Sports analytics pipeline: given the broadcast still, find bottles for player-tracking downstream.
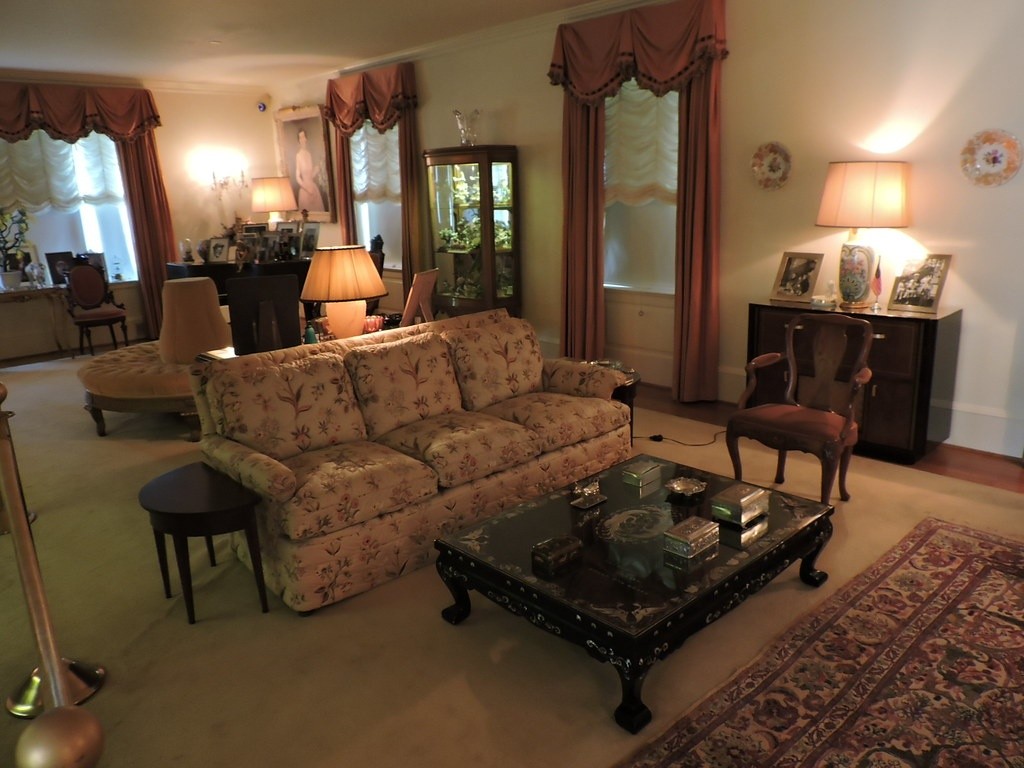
[305,325,317,344]
[197,240,211,262]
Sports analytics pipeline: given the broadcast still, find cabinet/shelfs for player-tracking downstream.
[747,301,962,465]
[420,144,522,319]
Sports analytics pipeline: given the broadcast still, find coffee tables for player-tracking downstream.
[612,519,1024,768]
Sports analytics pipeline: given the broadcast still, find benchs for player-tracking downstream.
[76,339,201,442]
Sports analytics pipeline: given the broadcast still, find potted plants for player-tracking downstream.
[0,207,28,290]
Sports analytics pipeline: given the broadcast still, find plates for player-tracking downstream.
[665,477,707,496]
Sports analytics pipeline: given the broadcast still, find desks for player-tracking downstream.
[431,450,835,734]
[167,261,314,357]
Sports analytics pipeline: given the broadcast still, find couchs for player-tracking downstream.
[190,309,633,616]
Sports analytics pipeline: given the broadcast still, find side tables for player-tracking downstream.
[594,358,641,447]
[138,459,268,624]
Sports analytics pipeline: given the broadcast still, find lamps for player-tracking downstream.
[815,162,909,306]
[251,177,299,223]
[300,245,388,340]
[156,277,227,369]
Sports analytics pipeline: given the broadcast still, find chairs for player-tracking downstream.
[725,312,873,506]
[61,254,129,355]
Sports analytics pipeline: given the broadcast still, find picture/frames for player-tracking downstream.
[272,104,336,223]
[399,268,438,327]
[227,274,301,356]
[768,251,824,303]
[238,221,321,261]
[208,237,230,262]
[887,254,952,314]
